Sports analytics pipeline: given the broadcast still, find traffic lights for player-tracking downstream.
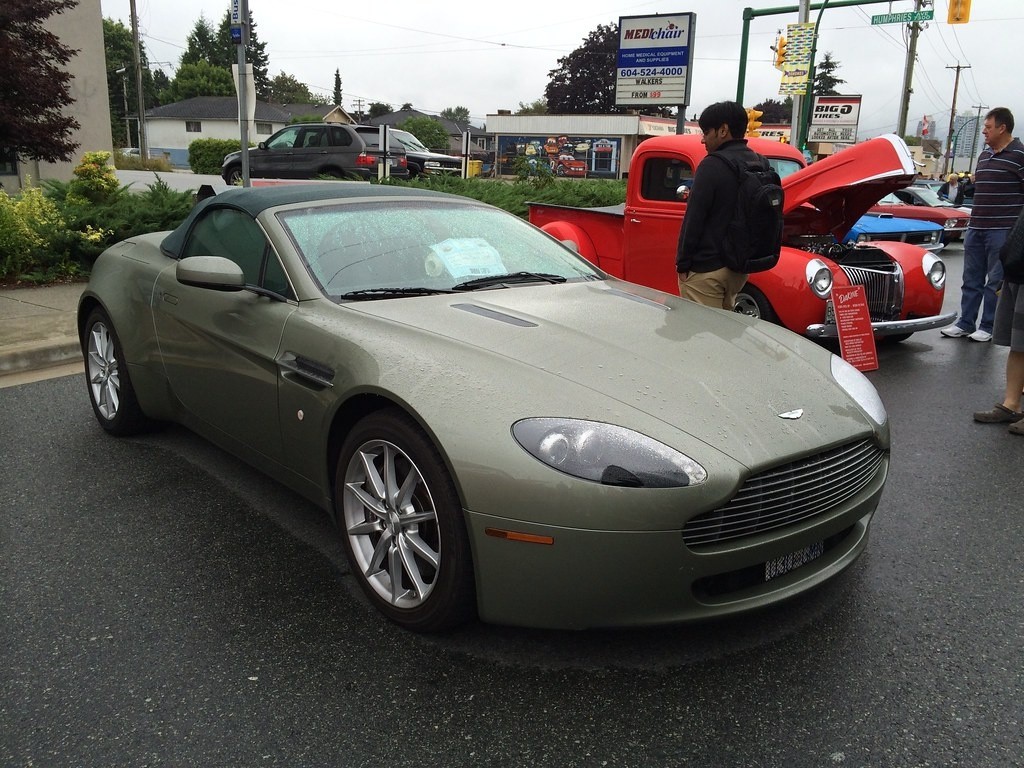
[747,110,763,138]
[775,35,788,70]
[780,137,786,142]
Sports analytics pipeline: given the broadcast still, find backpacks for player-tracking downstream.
[709,150,785,274]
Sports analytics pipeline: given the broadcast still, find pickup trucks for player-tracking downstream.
[522,132,959,340]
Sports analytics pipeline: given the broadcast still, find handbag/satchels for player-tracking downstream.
[996,204,1024,286]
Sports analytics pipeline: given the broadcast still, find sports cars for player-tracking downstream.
[76,181,893,635]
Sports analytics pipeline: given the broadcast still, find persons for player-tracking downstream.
[910,171,975,197]
[941,107,1024,344]
[937,174,964,205]
[974,209,1024,435]
[676,101,784,311]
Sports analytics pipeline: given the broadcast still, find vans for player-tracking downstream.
[947,173,972,182]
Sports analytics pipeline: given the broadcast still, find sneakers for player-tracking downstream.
[941,325,975,338]
[966,330,992,341]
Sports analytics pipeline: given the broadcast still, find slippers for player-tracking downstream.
[1008,417,1024,436]
[973,401,1023,421]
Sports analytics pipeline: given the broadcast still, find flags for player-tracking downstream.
[923,115,929,135]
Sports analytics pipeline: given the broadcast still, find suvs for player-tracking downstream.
[221,121,410,186]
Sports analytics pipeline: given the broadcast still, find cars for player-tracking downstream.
[912,179,973,204]
[119,148,151,159]
[834,216,944,256]
[867,193,970,248]
[894,187,972,226]
[307,126,462,178]
